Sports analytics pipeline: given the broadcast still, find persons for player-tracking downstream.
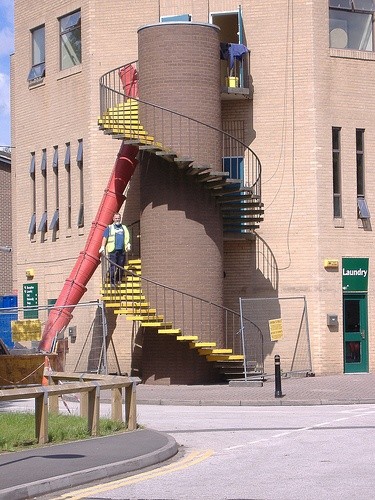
[98,213,131,287]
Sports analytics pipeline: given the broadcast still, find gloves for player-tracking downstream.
[126,243,131,251]
[99,245,105,253]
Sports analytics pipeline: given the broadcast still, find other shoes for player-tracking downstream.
[117,280,122,284]
[112,284,115,287]
